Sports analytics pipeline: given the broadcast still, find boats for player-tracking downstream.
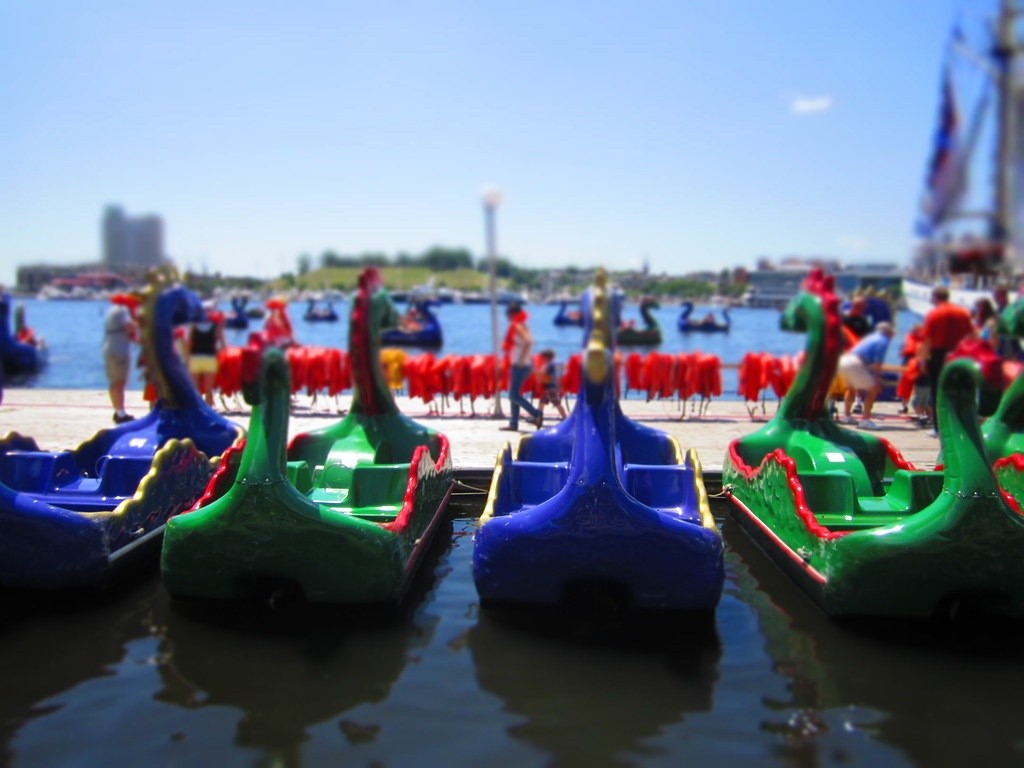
[1,266,902,388]
[722,263,1023,625]
[160,267,452,629]
[0,267,247,614]
[472,265,727,629]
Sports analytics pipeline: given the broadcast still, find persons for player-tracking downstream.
[499,303,544,431]
[839,322,897,429]
[100,286,145,424]
[704,312,714,322]
[525,348,569,426]
[897,284,1024,437]
[184,310,228,406]
[623,318,637,333]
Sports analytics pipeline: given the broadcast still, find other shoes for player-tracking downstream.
[113,413,133,423]
[535,416,542,430]
[841,416,858,424]
[858,420,881,429]
[526,417,536,423]
[500,426,517,431]
